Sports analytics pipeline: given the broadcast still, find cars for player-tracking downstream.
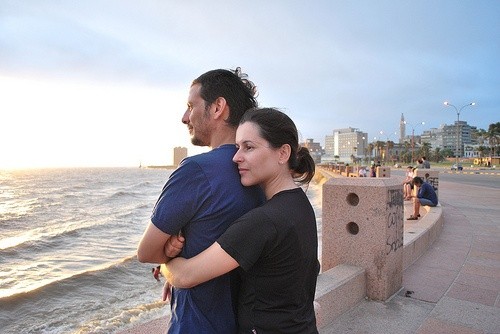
[451,165,462,171]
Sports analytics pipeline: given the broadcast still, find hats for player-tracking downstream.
[408,166,413,168]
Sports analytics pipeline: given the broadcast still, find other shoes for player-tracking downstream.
[407,217,418,220]
[411,214,420,217]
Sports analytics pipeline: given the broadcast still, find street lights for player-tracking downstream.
[403,122,425,166]
[444,102,476,165]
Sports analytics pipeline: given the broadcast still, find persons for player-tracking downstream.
[407,176,438,220]
[359,156,430,201]
[154,265,163,281]
[159,106,321,334]
[137,66,267,334]
